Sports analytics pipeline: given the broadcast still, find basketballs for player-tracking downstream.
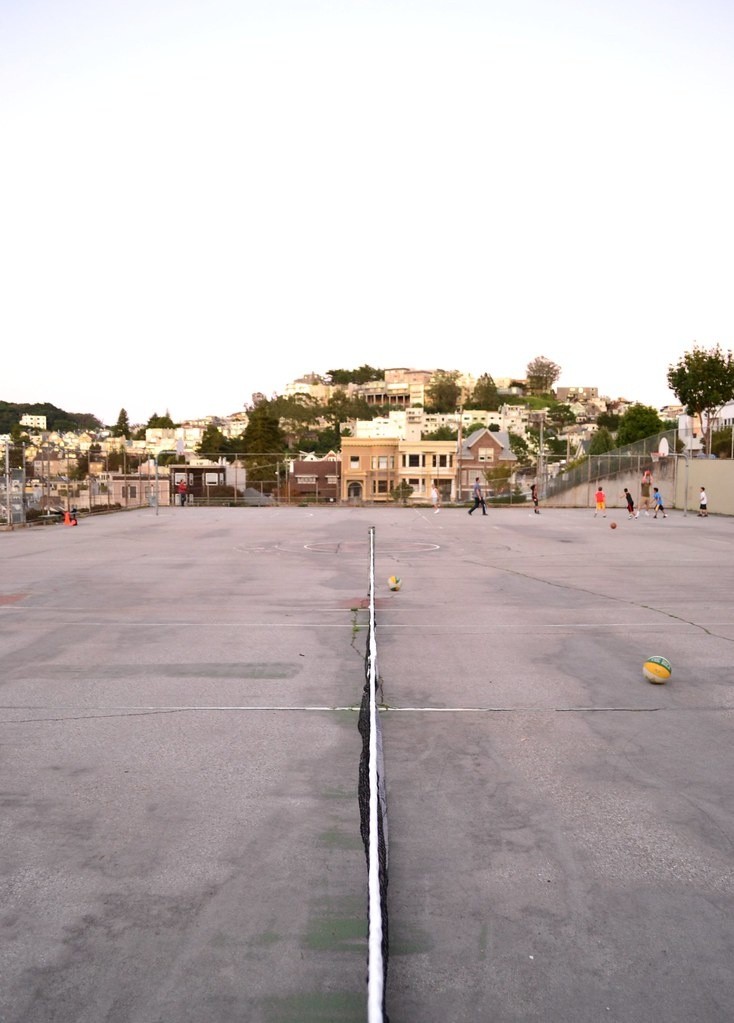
[611,522,616,529]
[643,656,672,685]
[645,471,651,476]
[387,576,402,591]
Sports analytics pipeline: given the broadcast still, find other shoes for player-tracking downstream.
[482,513,488,515]
[636,511,640,517]
[468,511,473,516]
[703,514,708,517]
[663,515,667,518]
[602,514,606,517]
[435,508,440,513]
[697,514,702,517]
[634,516,638,520]
[644,510,649,516]
[628,516,631,520]
[652,515,657,518]
[534,510,541,514]
[593,513,597,517]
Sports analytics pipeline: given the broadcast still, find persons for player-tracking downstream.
[431,484,440,514]
[621,488,636,519]
[530,485,539,514]
[636,471,653,516]
[697,487,708,518]
[651,488,667,518]
[179,479,188,506]
[595,487,606,518]
[468,477,488,516]
